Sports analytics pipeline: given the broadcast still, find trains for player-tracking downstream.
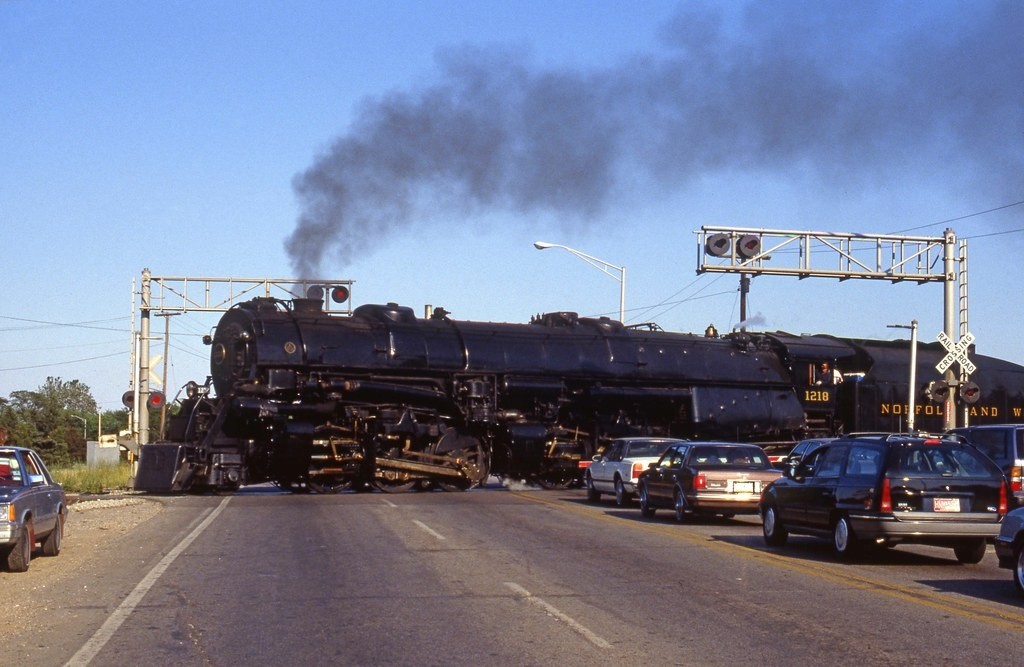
[134,294,1022,493]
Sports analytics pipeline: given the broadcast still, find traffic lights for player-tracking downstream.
[307,285,349,303]
[122,388,166,409]
[707,232,761,258]
[927,379,981,404]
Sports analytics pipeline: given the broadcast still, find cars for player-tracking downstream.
[586,436,688,505]
[0,446,67,573]
[636,441,783,523]
[781,438,840,474]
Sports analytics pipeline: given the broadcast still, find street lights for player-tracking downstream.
[71,414,87,440]
[534,241,626,325]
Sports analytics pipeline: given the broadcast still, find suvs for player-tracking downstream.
[759,431,1009,565]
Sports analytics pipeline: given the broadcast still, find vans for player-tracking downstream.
[937,424,1023,511]
